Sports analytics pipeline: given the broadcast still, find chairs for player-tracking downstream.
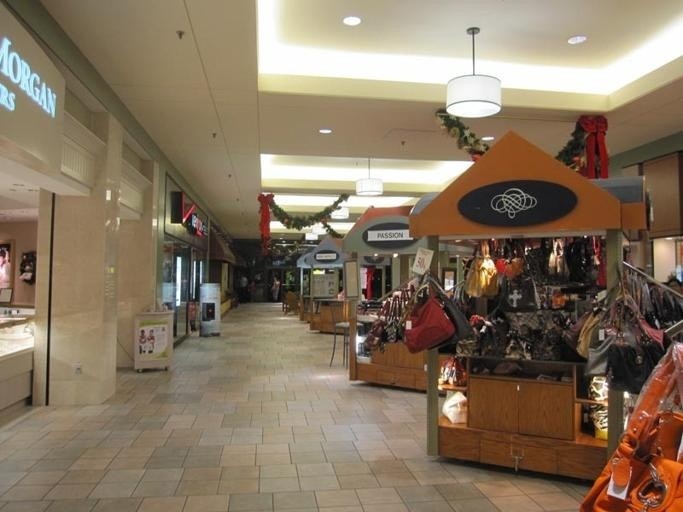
[281,290,301,314]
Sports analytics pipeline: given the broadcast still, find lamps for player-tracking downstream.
[445,27,502,118]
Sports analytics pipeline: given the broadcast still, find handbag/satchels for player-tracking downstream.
[403,273,474,355]
[456,236,683,392]
[579,343,683,511]
[365,279,416,350]
[440,390,468,425]
[438,357,466,387]
